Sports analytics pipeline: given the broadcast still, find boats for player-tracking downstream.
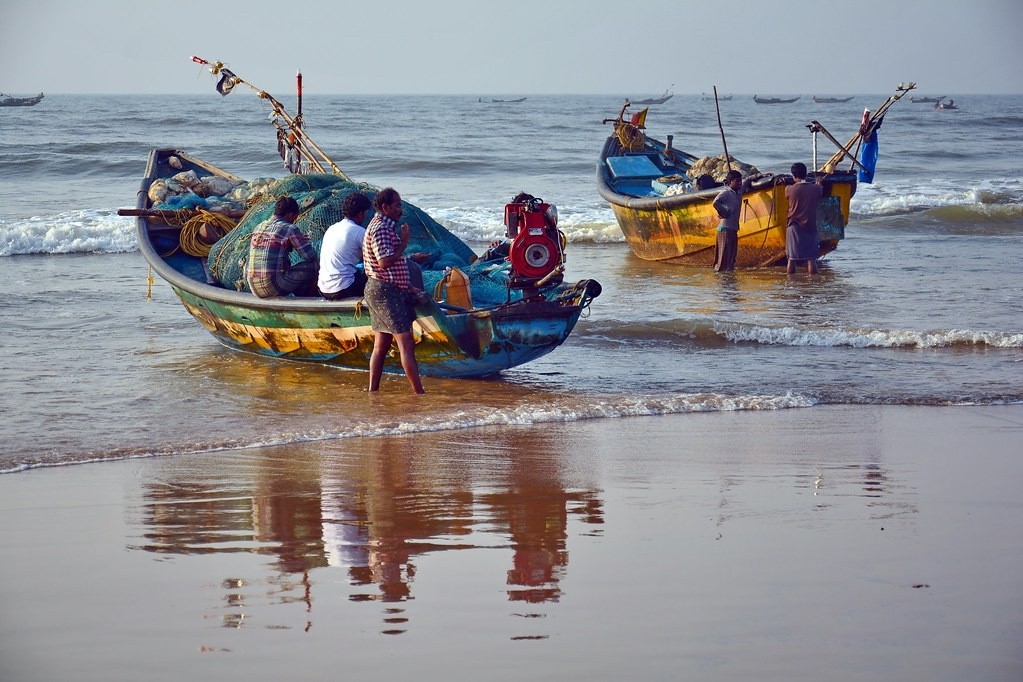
[594,78,922,268]
[811,96,856,103]
[492,97,528,103]
[935,99,959,110]
[0,92,47,108]
[703,94,734,101]
[115,53,605,380]
[752,94,802,104]
[909,96,949,104]
[625,90,676,105]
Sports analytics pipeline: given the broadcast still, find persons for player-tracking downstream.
[315,195,433,302]
[784,162,825,275]
[249,197,320,299]
[712,170,774,273]
[363,188,427,395]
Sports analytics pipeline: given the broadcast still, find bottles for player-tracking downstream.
[437,266,473,314]
